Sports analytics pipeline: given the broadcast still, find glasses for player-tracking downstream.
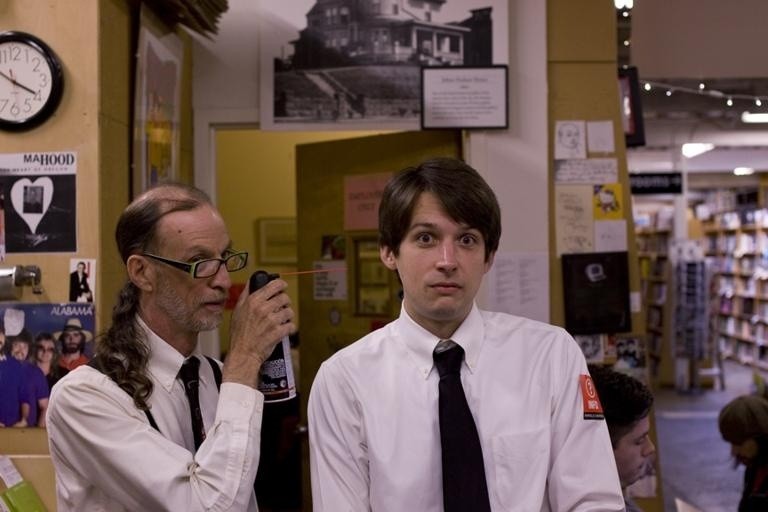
[140,250,249,279]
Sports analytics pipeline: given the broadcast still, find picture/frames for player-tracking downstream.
[420,65,508,130]
[257,216,298,265]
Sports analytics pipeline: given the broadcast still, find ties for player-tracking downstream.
[176,356,207,452]
[432,346,490,512]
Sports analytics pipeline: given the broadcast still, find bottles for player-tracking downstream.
[247,270,297,403]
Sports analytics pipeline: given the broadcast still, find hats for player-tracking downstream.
[719,396,768,440]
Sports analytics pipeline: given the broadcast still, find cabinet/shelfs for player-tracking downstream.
[637,225,768,393]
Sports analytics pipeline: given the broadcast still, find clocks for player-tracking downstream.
[0,30,64,133]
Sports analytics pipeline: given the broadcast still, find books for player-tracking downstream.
[635,184,768,391]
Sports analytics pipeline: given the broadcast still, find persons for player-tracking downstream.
[717,394,768,512]
[306,157,625,512]
[0,319,90,430]
[589,360,657,512]
[70,262,94,302]
[45,181,296,511]
